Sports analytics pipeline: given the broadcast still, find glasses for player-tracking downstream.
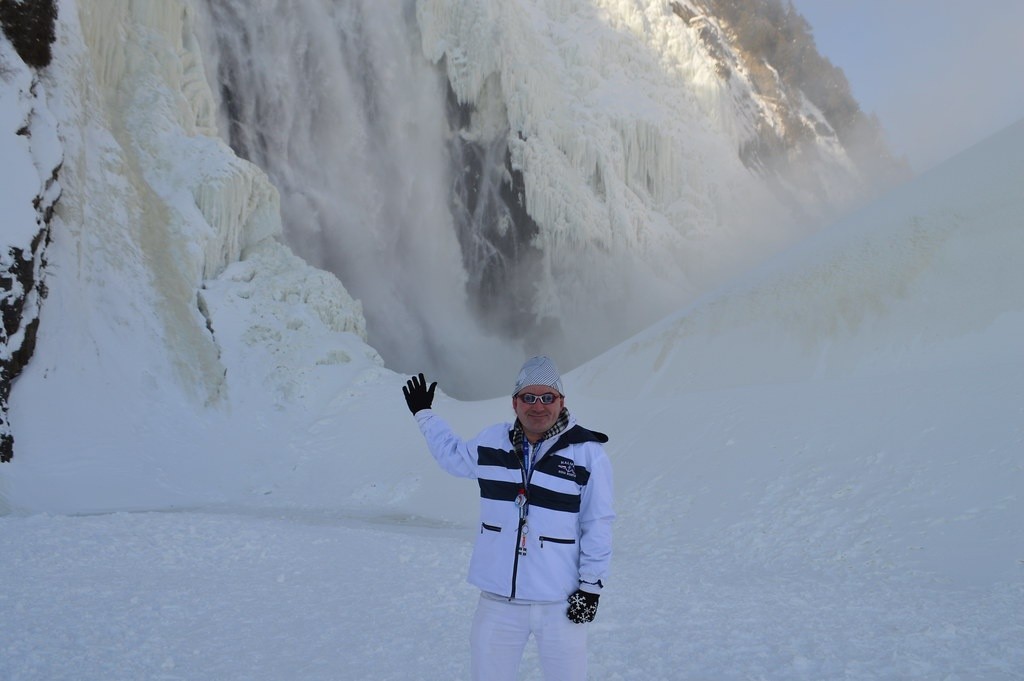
[517,393,560,404]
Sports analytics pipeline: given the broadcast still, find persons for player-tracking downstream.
[402,354,616,681]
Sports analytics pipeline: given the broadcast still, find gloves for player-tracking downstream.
[403,372,437,416]
[567,590,600,623]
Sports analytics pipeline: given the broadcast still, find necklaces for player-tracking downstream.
[514,435,542,556]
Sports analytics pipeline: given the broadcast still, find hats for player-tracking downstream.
[512,355,565,397]
